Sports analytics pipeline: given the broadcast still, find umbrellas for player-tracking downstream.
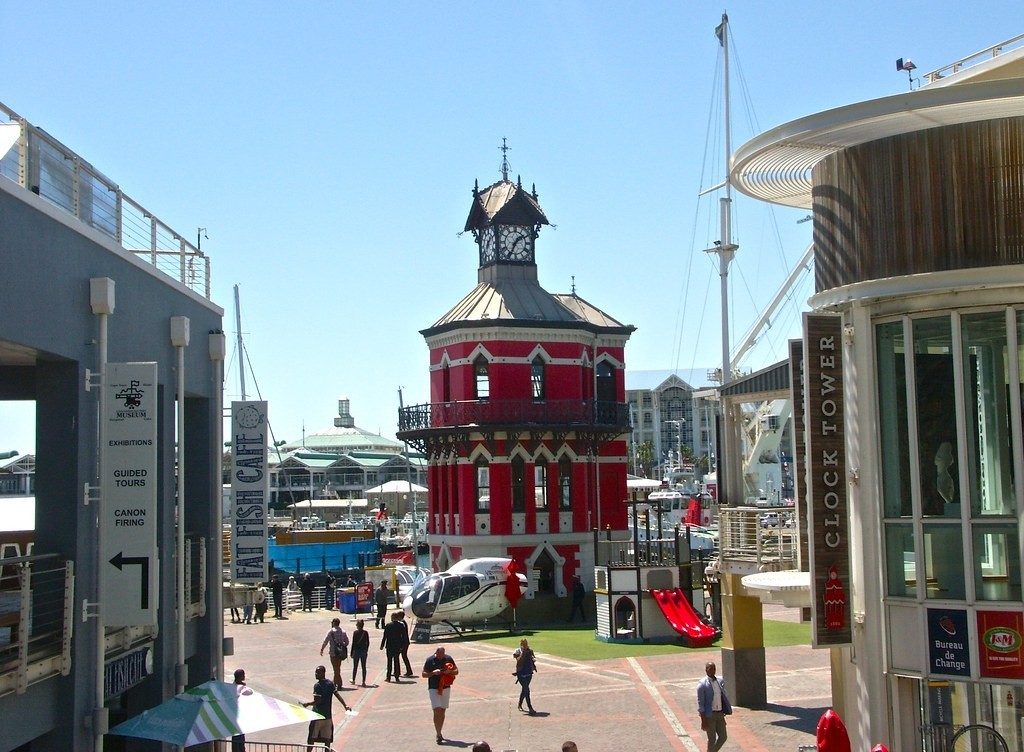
[104,678,325,752]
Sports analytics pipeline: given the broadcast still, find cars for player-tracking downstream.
[334,518,413,530]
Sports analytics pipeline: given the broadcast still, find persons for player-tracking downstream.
[232,570,355,624]
[514,638,537,714]
[935,442,954,504]
[375,580,389,629]
[351,620,369,686]
[422,646,458,745]
[380,612,405,682]
[473,740,492,752]
[392,612,413,676]
[566,575,586,622]
[320,618,349,689]
[563,741,578,752]
[303,666,351,752]
[697,662,727,752]
[231,669,245,752]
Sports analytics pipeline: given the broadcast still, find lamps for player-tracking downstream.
[896,57,920,91]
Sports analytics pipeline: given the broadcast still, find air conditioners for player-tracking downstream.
[763,414,777,433]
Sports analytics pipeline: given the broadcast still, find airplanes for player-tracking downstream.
[410,557,540,643]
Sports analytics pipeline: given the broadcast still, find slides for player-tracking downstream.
[649,587,716,639]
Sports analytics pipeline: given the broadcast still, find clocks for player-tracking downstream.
[497,223,533,263]
[480,225,497,265]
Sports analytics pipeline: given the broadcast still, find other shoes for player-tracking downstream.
[337,686,342,690]
[404,672,412,676]
[529,710,536,714]
[350,679,354,684]
[383,626,386,629]
[396,678,399,682]
[384,678,389,682]
[436,734,442,743]
[518,705,523,711]
[375,623,379,629]
[362,683,365,687]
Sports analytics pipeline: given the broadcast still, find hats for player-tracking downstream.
[331,618,340,623]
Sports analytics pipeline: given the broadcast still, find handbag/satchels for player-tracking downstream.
[335,643,347,659]
